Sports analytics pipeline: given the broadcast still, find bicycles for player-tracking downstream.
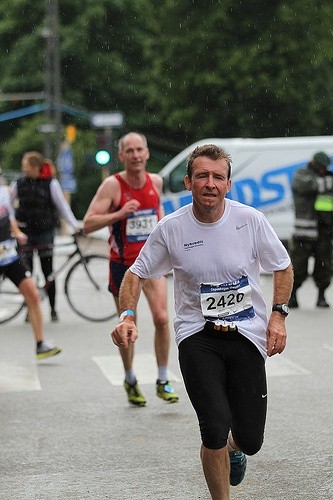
[0,229,118,324]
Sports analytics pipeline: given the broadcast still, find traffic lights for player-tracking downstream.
[96,149,111,165]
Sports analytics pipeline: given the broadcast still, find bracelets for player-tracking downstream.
[119,309,136,323]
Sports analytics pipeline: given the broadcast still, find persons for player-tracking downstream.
[289,152,333,309]
[82,132,180,406]
[6,151,84,322]
[109,144,293,500]
[0,164,60,360]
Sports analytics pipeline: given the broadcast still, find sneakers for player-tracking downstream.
[155,379,179,403]
[228,449,246,487]
[36,345,61,360]
[123,376,146,407]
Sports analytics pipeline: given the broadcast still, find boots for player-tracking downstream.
[317,289,330,306]
[288,287,298,307]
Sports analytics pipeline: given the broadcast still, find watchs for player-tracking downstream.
[272,303,290,317]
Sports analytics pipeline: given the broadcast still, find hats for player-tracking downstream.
[313,152,331,165]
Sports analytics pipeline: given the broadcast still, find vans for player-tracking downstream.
[156,135,332,245]
